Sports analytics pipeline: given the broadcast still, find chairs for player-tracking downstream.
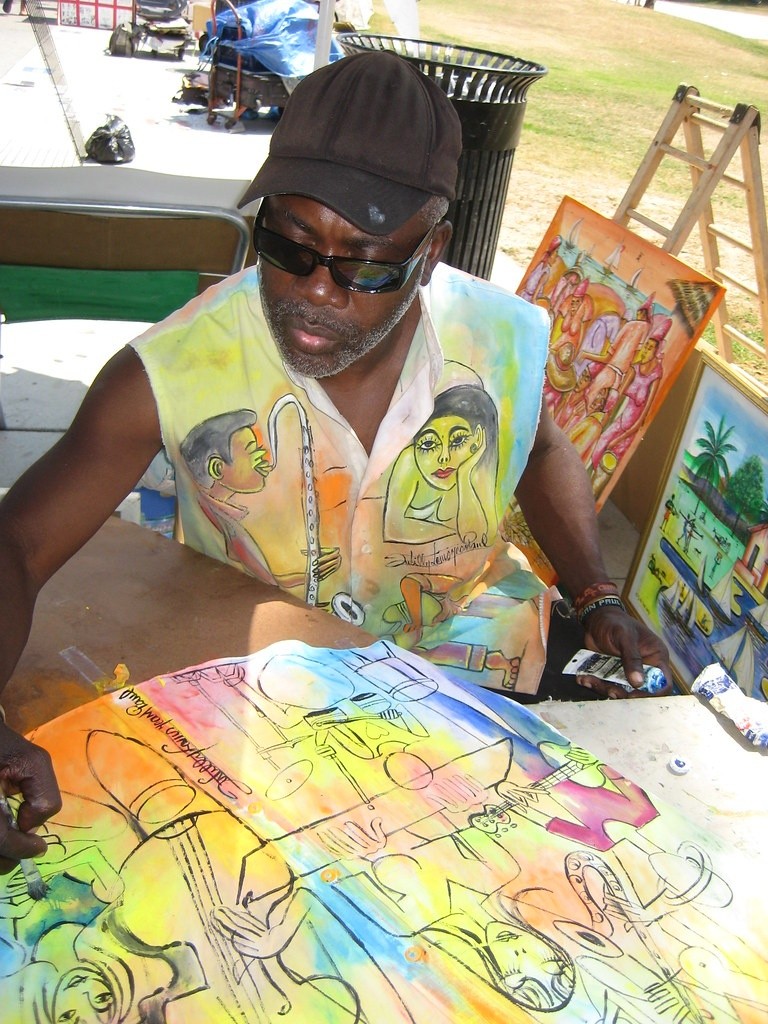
[0,198,253,344]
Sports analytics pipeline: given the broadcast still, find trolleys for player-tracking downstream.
[132,0,187,61]
[207,0,248,130]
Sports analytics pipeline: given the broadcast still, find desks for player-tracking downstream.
[1,491,683,1024]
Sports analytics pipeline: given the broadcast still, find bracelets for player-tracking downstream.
[571,582,622,624]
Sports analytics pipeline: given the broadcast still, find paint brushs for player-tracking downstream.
[0,784,52,902]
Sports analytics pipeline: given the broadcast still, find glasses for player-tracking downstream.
[253,196,436,294]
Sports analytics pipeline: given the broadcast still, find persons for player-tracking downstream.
[0,49,675,878]
[2,0,27,16]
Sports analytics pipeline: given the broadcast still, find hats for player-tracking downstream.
[238,50,463,236]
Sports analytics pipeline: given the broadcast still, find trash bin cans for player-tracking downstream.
[338,31,550,281]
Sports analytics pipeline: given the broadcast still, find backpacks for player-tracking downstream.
[109,23,135,57]
